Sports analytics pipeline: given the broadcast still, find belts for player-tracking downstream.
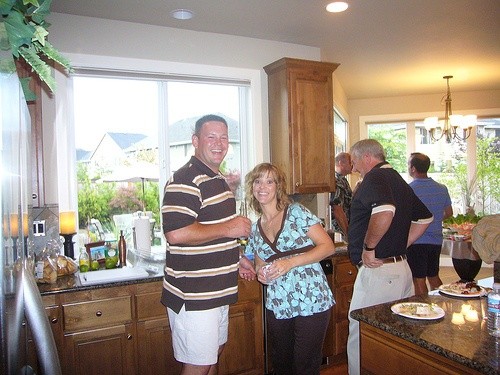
[357,254,406,269]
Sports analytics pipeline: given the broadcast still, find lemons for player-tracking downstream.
[79,258,116,272]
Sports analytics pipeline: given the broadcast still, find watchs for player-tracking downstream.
[362,242,375,251]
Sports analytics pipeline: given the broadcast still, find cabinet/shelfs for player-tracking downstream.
[263,57,341,193]
[6,253,359,375]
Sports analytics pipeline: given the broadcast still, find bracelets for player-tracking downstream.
[239,254,245,260]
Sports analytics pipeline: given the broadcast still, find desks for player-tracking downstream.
[350,276,500,375]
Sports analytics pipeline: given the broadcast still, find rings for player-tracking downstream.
[277,270,280,274]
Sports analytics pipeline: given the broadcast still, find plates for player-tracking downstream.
[439,283,486,298]
[391,303,445,320]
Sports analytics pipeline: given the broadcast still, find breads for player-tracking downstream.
[33,255,78,283]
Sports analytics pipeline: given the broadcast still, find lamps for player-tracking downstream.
[423,76,476,142]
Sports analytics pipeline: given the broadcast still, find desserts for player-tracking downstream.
[456,279,480,293]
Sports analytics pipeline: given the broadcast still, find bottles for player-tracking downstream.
[488,283,500,338]
[77,230,128,272]
[238,201,249,245]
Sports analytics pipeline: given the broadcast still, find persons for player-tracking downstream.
[160,115,256,375]
[241,163,336,375]
[346,138,434,375]
[329,151,354,244]
[407,153,453,296]
